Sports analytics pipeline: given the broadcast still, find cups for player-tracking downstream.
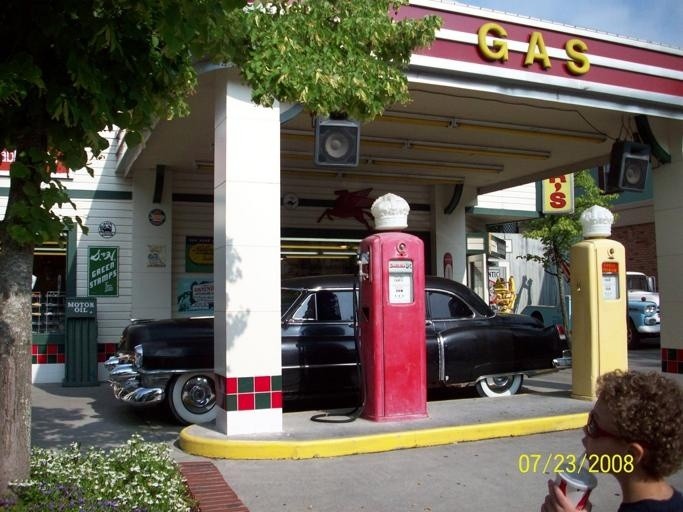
[555,463,598,512]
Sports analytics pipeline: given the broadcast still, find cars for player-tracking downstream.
[105,274,573,425]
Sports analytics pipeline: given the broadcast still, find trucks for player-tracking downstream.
[520,271,661,350]
[179,282,214,311]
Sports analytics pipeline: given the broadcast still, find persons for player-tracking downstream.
[541,368,683,512]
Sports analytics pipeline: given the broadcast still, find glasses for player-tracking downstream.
[583,415,619,442]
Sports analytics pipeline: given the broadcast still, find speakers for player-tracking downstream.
[314,111,361,167]
[607,137,651,193]
